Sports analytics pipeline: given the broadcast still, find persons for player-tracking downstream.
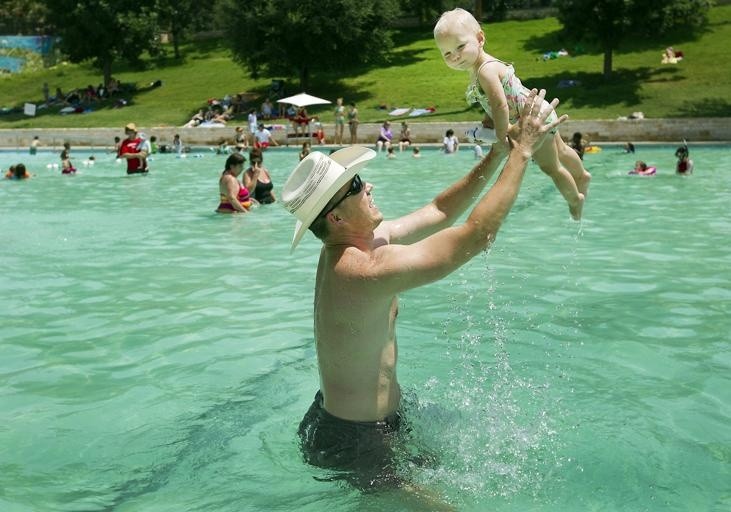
[661,47,684,63]
[280,86,571,512]
[627,158,654,177]
[215,151,254,217]
[536,46,571,60]
[673,148,692,175]
[241,147,278,206]
[433,8,592,219]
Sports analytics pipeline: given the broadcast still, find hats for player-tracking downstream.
[124,123,138,134]
[276,144,381,257]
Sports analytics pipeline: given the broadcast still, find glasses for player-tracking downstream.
[322,172,367,219]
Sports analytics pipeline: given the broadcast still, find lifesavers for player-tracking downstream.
[584,146,601,154]
[628,166,656,176]
[318,129,325,142]
